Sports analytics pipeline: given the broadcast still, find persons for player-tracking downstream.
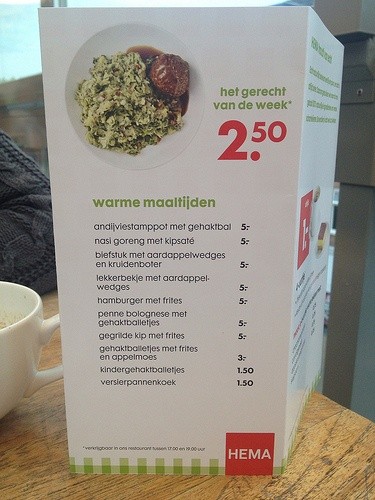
[0,127,56,298]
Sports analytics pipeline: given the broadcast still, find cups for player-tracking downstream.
[1,281,67,421]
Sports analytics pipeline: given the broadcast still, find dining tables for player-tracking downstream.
[0,280,375,500]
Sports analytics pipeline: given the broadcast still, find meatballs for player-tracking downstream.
[150,54,190,98]
